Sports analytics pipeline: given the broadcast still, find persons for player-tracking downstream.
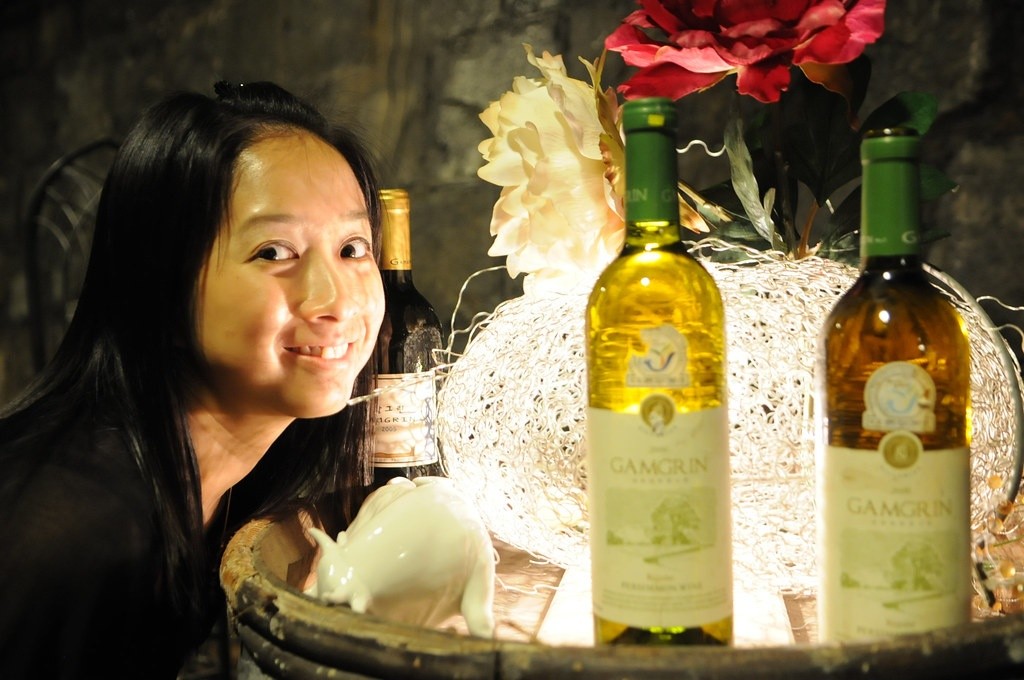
[0,82,388,680]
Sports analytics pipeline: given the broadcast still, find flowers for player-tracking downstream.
[476,0,937,295]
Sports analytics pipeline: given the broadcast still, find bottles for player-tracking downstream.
[816,128,973,642]
[340,189,445,486]
[584,98,734,644]
